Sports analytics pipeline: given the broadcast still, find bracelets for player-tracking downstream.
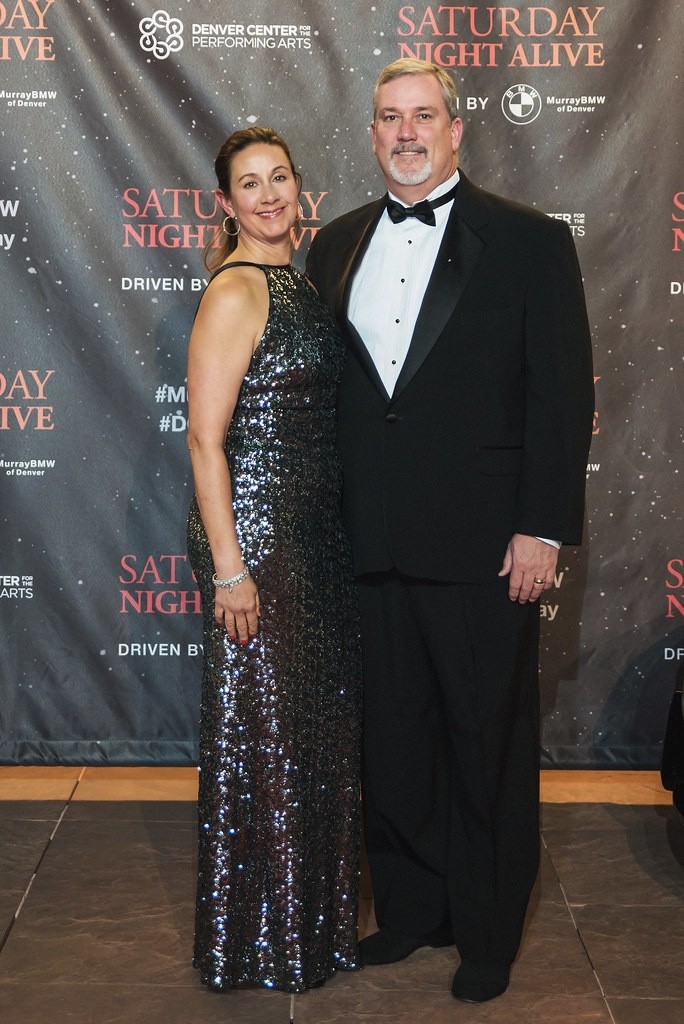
[213,565,250,593]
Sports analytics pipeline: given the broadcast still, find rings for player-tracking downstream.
[534,578,546,584]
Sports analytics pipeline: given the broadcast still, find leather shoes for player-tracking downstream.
[451,960,511,1004]
[355,925,424,966]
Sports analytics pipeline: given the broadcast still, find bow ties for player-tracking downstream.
[384,180,460,226]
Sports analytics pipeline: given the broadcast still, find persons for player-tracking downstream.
[308,59,594,1001]
[185,128,361,991]
